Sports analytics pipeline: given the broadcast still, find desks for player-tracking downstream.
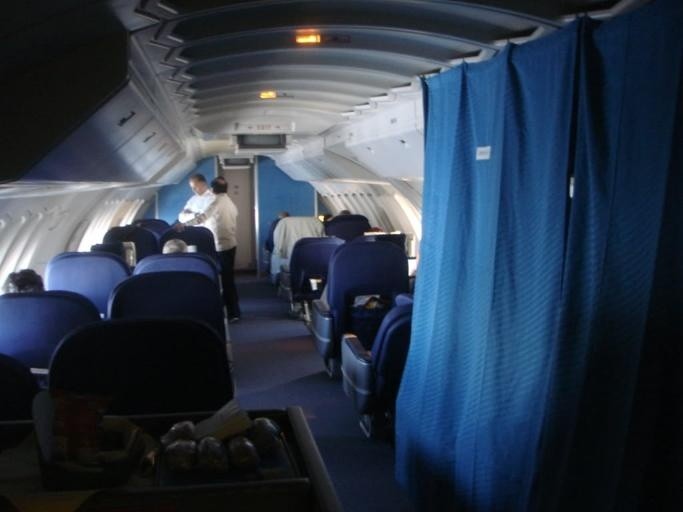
[0,407,344,512]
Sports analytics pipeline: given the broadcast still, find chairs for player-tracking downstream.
[308,236,408,378]
[339,302,413,437]
[159,224,216,252]
[103,225,154,259]
[269,215,325,285]
[275,236,346,306]
[134,217,169,234]
[132,252,219,288]
[0,352,41,450]
[325,215,370,240]
[47,313,235,418]
[0,289,100,386]
[44,252,129,318]
[105,270,228,346]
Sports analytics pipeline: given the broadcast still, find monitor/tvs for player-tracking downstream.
[224,159,249,166]
[236,135,286,149]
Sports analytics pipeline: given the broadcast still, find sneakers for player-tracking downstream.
[228,317,241,326]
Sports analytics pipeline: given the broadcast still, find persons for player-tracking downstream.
[3,269,43,294]
[162,238,188,254]
[178,174,216,228]
[173,176,240,323]
[268,212,289,250]
